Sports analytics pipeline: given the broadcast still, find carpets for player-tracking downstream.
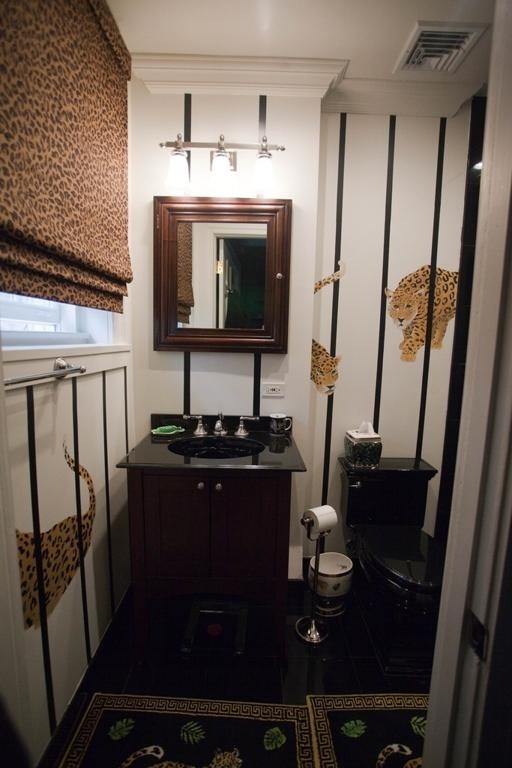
[40,691,316,767]
[305,692,431,767]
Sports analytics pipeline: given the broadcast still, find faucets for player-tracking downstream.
[212,410,229,437]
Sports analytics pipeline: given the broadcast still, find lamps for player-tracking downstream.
[158,131,287,193]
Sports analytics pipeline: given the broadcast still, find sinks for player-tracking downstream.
[167,436,267,460]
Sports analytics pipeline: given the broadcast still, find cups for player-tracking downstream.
[268,414,294,437]
[269,435,292,455]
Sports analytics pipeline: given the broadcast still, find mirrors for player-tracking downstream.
[150,192,293,355]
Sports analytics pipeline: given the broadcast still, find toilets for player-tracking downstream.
[336,454,439,665]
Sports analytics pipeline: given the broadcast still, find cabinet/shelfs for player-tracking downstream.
[115,424,308,646]
